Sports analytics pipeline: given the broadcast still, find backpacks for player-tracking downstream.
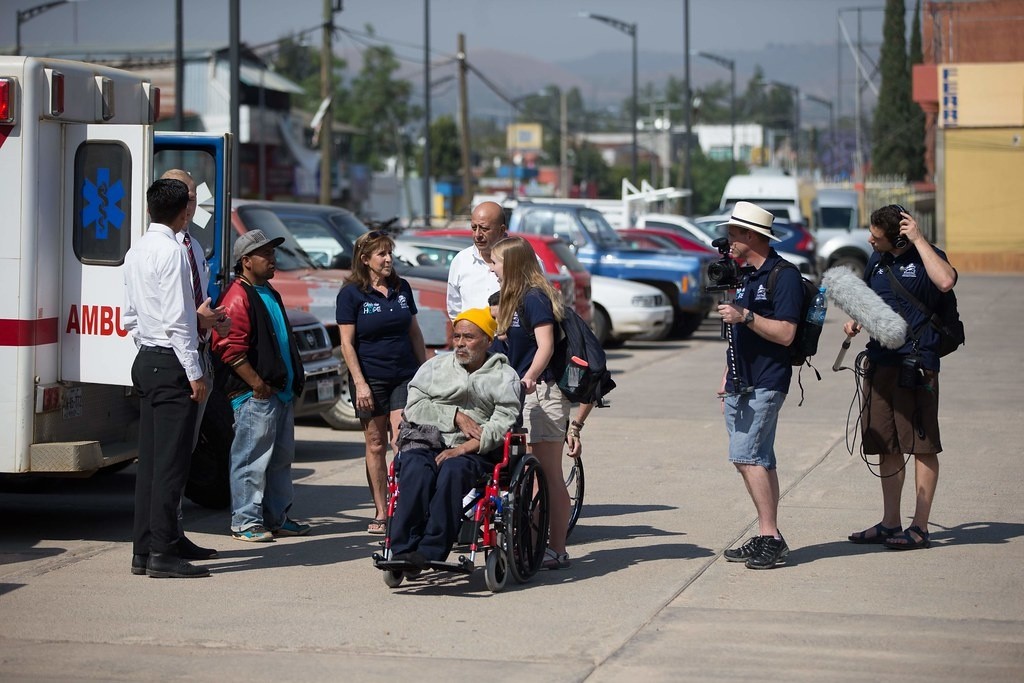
[560,306,617,403]
[765,258,828,368]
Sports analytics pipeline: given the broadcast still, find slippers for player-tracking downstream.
[367,517,388,534]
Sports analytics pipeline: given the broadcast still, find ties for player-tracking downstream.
[183,233,208,338]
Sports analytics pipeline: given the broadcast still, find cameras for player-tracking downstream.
[707,237,757,284]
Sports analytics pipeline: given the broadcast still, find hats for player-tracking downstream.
[452,306,498,341]
[715,201,782,243]
[232,229,286,261]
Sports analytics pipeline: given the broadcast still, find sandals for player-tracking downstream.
[884,525,930,550]
[848,521,903,544]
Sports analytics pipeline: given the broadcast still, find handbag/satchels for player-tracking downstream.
[883,250,965,359]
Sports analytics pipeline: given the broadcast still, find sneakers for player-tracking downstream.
[232,526,273,542]
[539,547,573,571]
[273,517,312,536]
[724,529,790,569]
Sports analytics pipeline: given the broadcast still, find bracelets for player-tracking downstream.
[567,421,584,436]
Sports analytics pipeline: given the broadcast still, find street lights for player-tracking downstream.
[801,93,835,182]
[689,49,737,175]
[759,79,800,168]
[422,0,457,226]
[578,12,639,195]
[11,0,70,56]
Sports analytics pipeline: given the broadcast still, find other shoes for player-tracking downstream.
[131,552,210,578]
[178,535,218,560]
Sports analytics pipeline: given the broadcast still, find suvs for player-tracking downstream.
[259,200,450,282]
[188,197,453,431]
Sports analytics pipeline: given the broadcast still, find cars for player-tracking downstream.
[294,172,878,350]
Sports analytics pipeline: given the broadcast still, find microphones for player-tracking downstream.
[822,264,909,350]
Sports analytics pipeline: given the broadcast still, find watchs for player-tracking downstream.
[745,310,754,325]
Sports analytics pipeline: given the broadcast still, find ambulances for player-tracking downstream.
[0,55,236,481]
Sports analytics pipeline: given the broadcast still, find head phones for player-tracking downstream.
[893,203,908,249]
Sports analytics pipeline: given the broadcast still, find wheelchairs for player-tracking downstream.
[371,381,586,593]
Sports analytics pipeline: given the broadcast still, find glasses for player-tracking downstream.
[361,230,389,255]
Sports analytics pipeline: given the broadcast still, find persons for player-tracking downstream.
[719,200,800,570]
[845,205,960,552]
[484,289,594,458]
[122,170,569,580]
